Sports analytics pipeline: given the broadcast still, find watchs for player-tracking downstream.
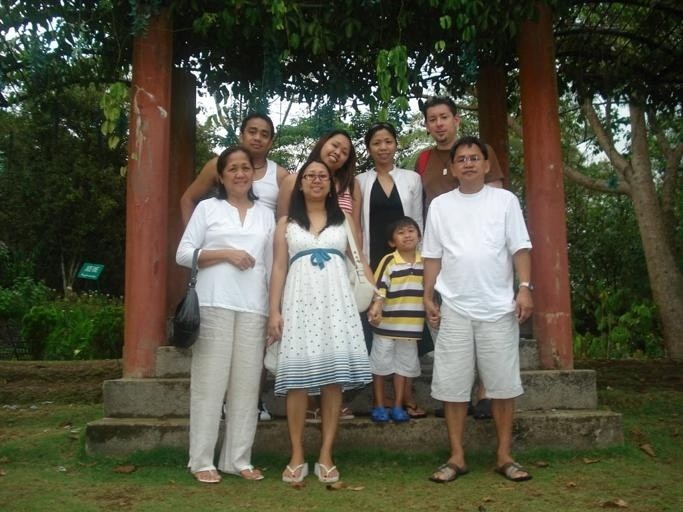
[517,280,536,293]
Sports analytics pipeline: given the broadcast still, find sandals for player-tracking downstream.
[194,469,221,483]
[240,465,264,480]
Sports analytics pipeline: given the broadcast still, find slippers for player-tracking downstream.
[372,405,426,421]
[304,407,322,424]
[430,461,468,483]
[496,460,532,481]
[340,407,354,421]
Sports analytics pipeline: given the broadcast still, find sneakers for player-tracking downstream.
[220,402,226,421]
[258,399,274,422]
[435,398,493,419]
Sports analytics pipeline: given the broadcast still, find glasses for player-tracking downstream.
[425,94,450,105]
[369,123,392,131]
[301,173,330,181]
[456,155,480,165]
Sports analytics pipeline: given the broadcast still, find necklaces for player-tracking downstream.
[247,157,267,175]
[434,147,456,185]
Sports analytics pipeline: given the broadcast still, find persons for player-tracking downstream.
[265,159,376,486]
[277,129,366,424]
[412,98,501,421]
[182,114,289,424]
[421,138,536,484]
[367,218,427,427]
[175,147,271,482]
[357,121,427,418]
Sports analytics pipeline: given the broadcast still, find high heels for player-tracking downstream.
[282,461,309,483]
[314,462,339,483]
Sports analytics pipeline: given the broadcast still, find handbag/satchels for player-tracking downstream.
[168,248,200,347]
[342,209,375,313]
